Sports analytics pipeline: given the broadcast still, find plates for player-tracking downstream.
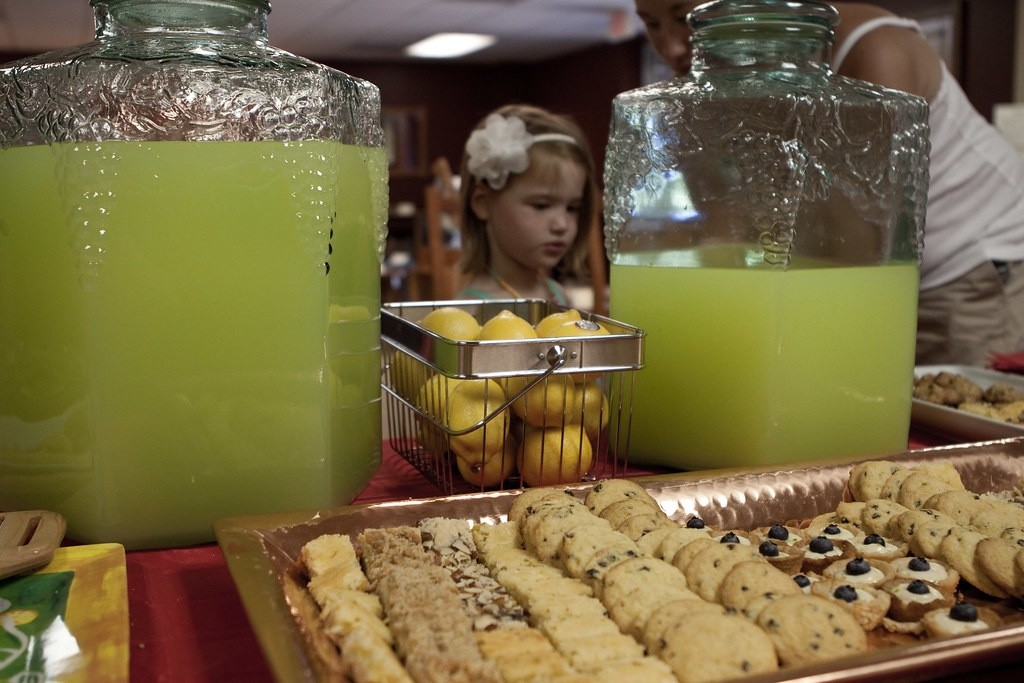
[215,436,1024,683]
[0,542,130,683]
[912,364,1024,442]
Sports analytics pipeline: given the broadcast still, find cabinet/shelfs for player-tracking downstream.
[408,182,605,313]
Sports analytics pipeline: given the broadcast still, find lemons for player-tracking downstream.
[391,307,614,490]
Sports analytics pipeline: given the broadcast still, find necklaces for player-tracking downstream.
[487,266,521,299]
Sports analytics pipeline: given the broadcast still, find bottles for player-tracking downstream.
[0,0,388,551]
[602,0,931,470]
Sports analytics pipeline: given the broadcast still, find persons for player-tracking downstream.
[635,0,1024,365]
[457,105,593,305]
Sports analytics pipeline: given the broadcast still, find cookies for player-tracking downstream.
[910,371,1024,427]
[295,457,1024,683]
